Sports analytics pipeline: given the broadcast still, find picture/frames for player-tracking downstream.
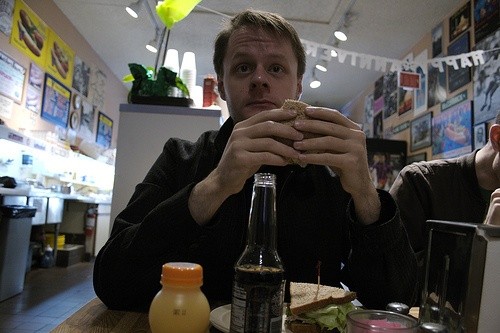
[373,0,500,177]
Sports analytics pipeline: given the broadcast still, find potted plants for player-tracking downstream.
[122,0,201,108]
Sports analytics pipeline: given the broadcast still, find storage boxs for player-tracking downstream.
[55,243,85,267]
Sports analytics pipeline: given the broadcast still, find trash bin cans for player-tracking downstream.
[0,205,37,302]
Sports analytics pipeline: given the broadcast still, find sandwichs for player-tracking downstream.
[285,282,364,333]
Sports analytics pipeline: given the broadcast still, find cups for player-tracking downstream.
[163,48,204,109]
[346,310,421,333]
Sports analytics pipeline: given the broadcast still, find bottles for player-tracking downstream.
[229,173,286,333]
[149,262,210,333]
[386,302,409,316]
[42,244,54,268]
[421,322,448,333]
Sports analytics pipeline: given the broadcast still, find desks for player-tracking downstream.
[0,186,112,261]
[46,296,228,333]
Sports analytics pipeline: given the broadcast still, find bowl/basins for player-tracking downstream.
[51,185,70,194]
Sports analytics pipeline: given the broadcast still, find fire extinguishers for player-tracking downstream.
[84,207,95,237]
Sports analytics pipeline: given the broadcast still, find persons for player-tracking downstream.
[91,11,424,322]
[471,42,500,112]
[384,112,500,308]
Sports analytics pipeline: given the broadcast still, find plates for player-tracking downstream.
[210,303,290,333]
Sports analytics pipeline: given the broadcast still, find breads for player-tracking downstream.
[272,100,315,167]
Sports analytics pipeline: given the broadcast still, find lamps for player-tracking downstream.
[307,39,339,89]
[126,0,146,20]
[145,28,161,53]
[333,12,353,41]
